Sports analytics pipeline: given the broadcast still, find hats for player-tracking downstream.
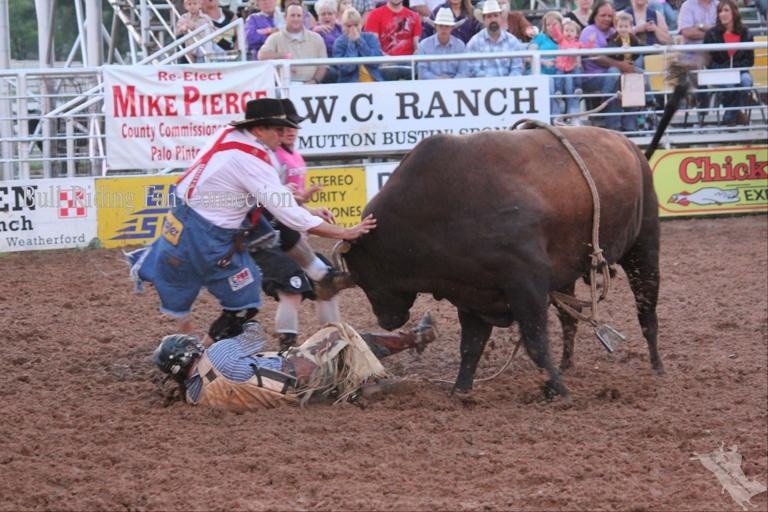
[425,7,468,29]
[473,0,509,24]
[282,98,305,124]
[231,98,302,130]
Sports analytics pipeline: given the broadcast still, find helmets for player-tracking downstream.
[153,334,206,380]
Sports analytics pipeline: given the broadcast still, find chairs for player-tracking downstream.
[750,35,768,123]
[644,54,693,126]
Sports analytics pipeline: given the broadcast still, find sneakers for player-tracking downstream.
[311,267,355,300]
[360,375,415,401]
[412,308,440,350]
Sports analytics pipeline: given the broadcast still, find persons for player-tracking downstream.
[622,0,677,67]
[243,99,341,352]
[336,1,351,22]
[126,99,376,349]
[332,8,381,83]
[257,4,330,84]
[417,7,467,79]
[551,18,596,103]
[420,1,483,45]
[563,0,596,31]
[579,2,641,131]
[678,1,721,116]
[705,1,755,127]
[365,1,422,81]
[497,1,538,44]
[352,0,376,30]
[245,0,284,62]
[311,1,343,58]
[527,12,580,127]
[153,308,439,413]
[201,0,239,55]
[601,10,659,107]
[174,0,218,64]
[408,0,446,44]
[466,0,525,78]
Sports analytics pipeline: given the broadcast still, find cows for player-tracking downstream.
[332,126,668,409]
